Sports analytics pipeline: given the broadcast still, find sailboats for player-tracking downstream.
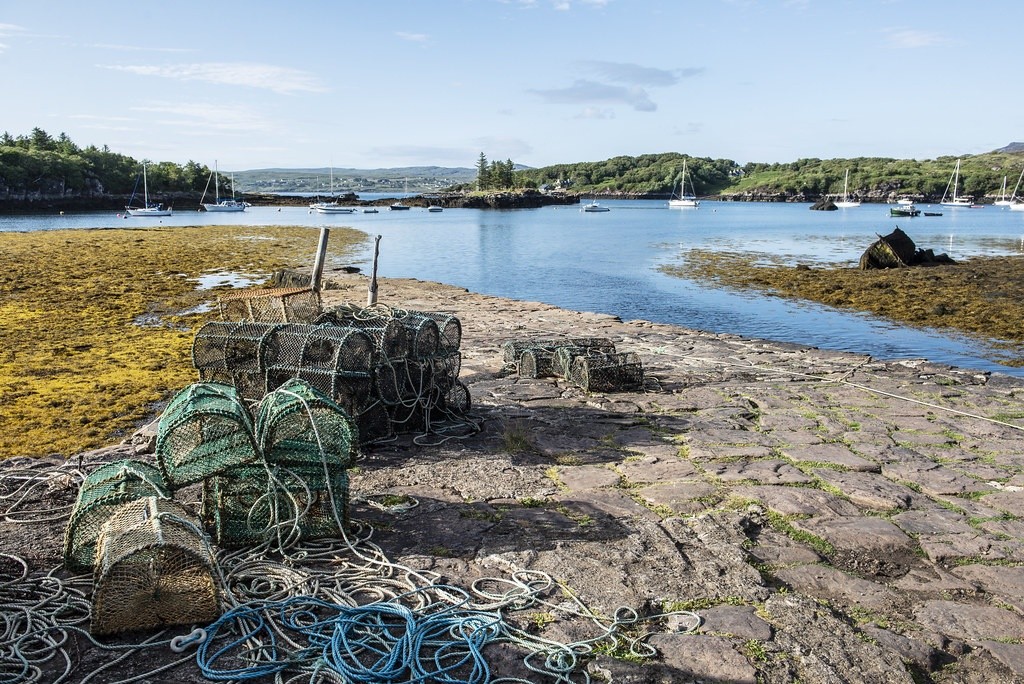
[834,167,861,207]
[125,160,173,216]
[309,177,338,209]
[993,176,1016,206]
[317,157,358,214]
[1009,169,1024,212]
[668,160,700,206]
[200,159,246,211]
[941,158,972,208]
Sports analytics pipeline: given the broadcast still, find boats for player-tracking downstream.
[955,196,975,204]
[428,205,443,212]
[364,209,379,213]
[890,205,921,218]
[242,200,252,207]
[390,198,409,210]
[924,212,943,216]
[897,198,913,205]
[584,200,610,212]
